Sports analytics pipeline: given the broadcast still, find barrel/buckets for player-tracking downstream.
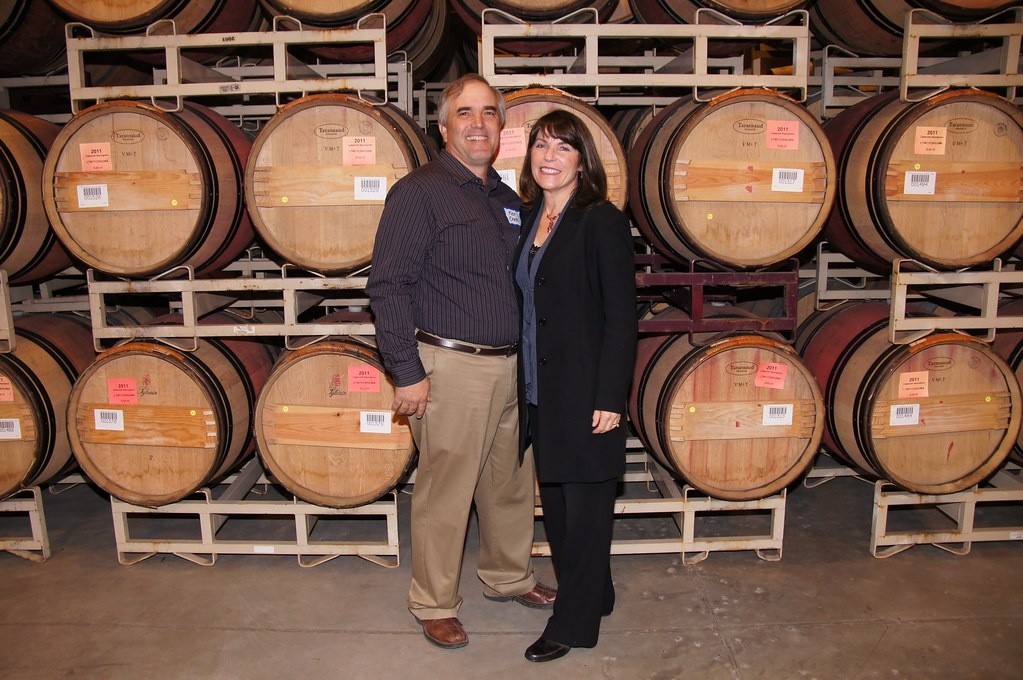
[0,306,287,506]
[808,0,1016,58]
[261,0,633,88]
[493,88,627,212]
[805,87,1023,278]
[604,87,837,273]
[42,99,254,281]
[0,0,272,114]
[738,287,1023,495]
[245,93,440,276]
[629,296,825,501]
[625,0,812,54]
[0,111,71,285]
[255,308,418,510]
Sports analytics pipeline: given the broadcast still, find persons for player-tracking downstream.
[366,72,559,649]
[512,109,637,663]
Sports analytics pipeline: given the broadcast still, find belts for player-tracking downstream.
[415,330,519,357]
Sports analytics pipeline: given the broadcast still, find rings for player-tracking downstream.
[613,423,619,427]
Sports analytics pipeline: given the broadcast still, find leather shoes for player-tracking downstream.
[525,635,571,661]
[416,616,469,648]
[483,581,557,610]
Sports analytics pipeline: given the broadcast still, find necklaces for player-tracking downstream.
[545,204,561,234]
